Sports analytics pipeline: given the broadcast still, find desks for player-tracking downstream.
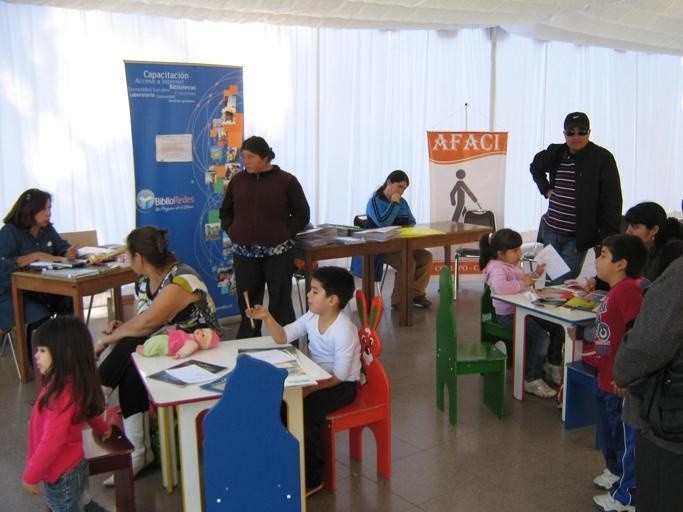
[293,221,493,327]
[493,287,606,416]
[10,257,144,383]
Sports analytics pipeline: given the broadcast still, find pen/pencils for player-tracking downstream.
[531,279,550,282]
[243,291,255,329]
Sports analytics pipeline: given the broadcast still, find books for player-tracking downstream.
[146,342,318,393]
[27,244,130,281]
[524,278,609,313]
[295,222,403,249]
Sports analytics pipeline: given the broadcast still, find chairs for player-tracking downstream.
[53,229,113,330]
[424,261,519,425]
[453,208,498,303]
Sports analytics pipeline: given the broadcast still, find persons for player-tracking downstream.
[477,228,565,400]
[244,266,361,499]
[607,257,682,511]
[92,225,225,489]
[20,315,107,512]
[218,136,311,350]
[136,328,220,359]
[530,110,623,287]
[567,322,598,344]
[586,227,620,292]
[366,170,433,309]
[593,232,649,512]
[0,189,81,332]
[584,200,683,293]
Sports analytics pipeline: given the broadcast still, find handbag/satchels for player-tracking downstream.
[639,371,683,443]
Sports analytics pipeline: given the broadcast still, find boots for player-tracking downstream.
[102,411,156,486]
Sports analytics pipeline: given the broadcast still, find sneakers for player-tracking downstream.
[524,378,558,398]
[544,361,562,385]
[592,468,621,490]
[412,295,432,308]
[591,493,635,512]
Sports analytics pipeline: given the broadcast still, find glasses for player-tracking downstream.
[566,128,588,136]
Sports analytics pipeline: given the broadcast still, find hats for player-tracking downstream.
[564,111,590,130]
